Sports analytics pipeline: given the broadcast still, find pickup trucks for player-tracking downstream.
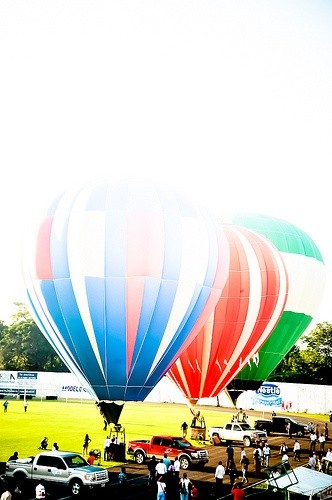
[209,421,268,448]
[253,415,310,437]
[5,451,110,496]
[126,434,209,469]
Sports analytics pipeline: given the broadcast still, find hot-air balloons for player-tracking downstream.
[164,226,289,440]
[228,211,328,412]
[21,170,231,458]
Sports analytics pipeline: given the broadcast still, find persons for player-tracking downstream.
[251,420,270,473]
[103,435,120,461]
[181,408,207,441]
[285,419,292,439]
[214,444,250,500]
[210,433,221,445]
[0,473,45,500]
[24,400,28,412]
[115,467,128,493]
[292,440,301,462]
[147,453,197,500]
[281,400,293,411]
[3,400,9,412]
[271,411,276,416]
[231,407,249,424]
[8,452,18,461]
[304,409,332,475]
[280,442,289,463]
[82,433,101,465]
[40,437,59,451]
